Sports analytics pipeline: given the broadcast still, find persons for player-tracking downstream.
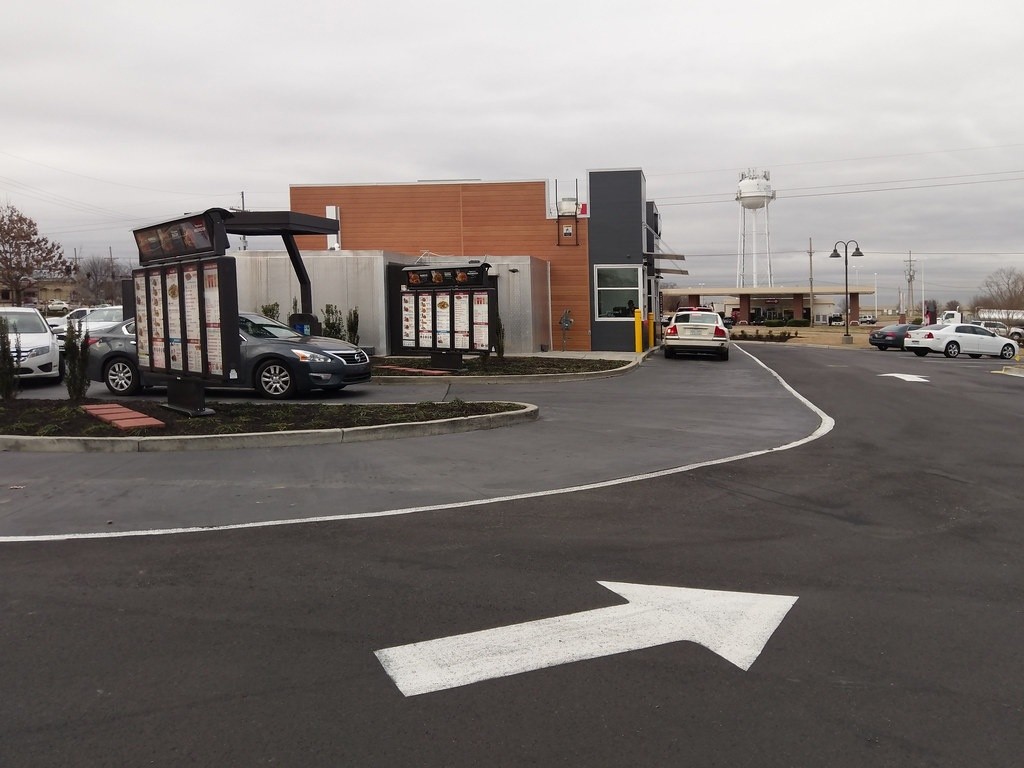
[624,299,639,317]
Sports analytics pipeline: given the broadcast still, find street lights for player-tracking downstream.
[830,239,864,337]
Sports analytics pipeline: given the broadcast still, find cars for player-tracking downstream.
[823,296,1023,360]
[656,279,788,361]
[1,279,376,406]
